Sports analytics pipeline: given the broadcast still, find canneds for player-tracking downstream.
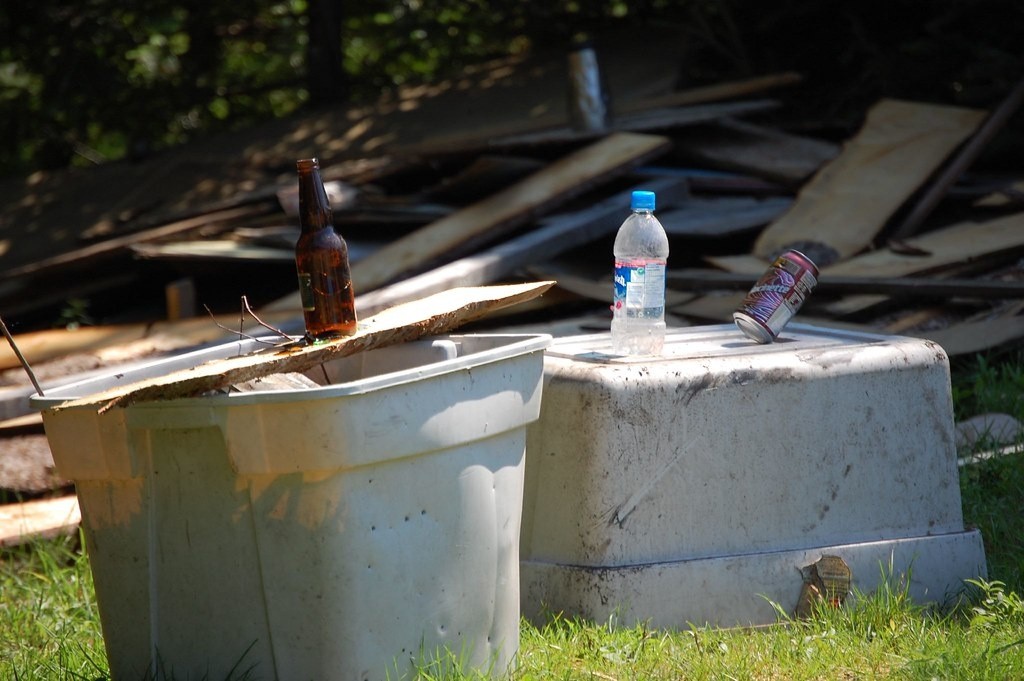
[732,249,819,344]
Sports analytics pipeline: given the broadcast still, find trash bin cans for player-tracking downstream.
[27,322,553,681]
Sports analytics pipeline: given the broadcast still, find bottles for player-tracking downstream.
[611,190,668,358]
[295,157,356,337]
[566,3,602,135]
